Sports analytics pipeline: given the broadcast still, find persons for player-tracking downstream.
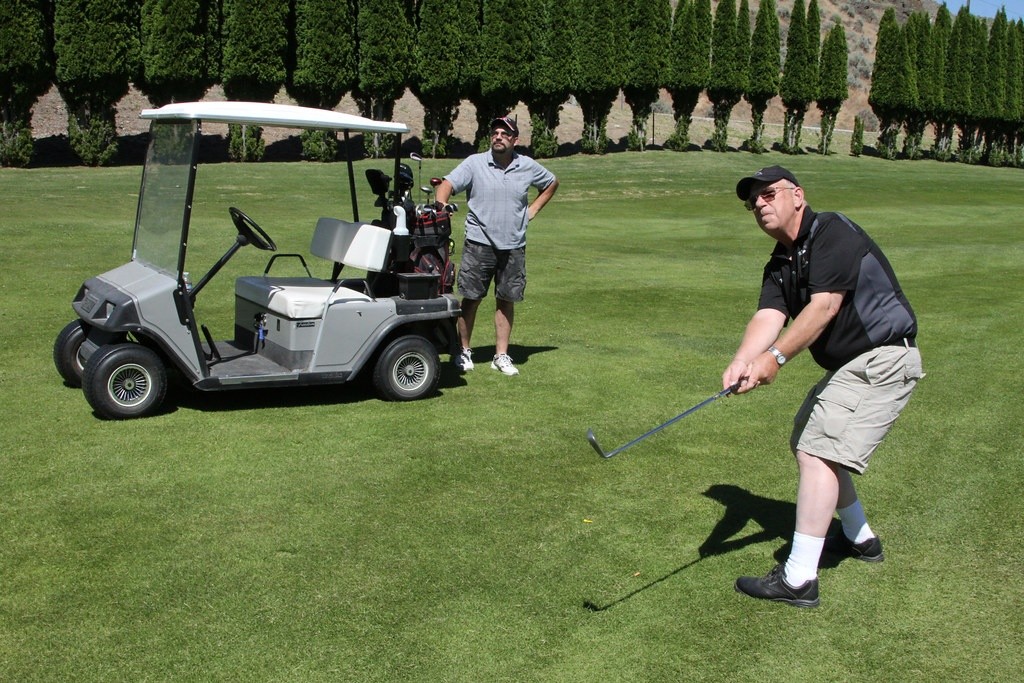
[435,115,560,375]
[719,163,927,609]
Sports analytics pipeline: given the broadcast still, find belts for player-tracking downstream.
[875,335,917,347]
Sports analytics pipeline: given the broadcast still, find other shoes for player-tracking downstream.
[735,564,820,610]
[825,526,884,562]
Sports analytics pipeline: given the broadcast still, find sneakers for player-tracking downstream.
[490,353,520,376]
[447,345,474,375]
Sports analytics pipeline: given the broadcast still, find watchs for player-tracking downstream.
[768,346,787,367]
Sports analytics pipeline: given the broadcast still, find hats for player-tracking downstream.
[491,116,519,136]
[736,165,800,201]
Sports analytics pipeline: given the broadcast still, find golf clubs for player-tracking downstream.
[401,153,460,220]
[586,380,747,462]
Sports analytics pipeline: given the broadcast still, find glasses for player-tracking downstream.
[744,187,794,211]
[490,131,512,137]
